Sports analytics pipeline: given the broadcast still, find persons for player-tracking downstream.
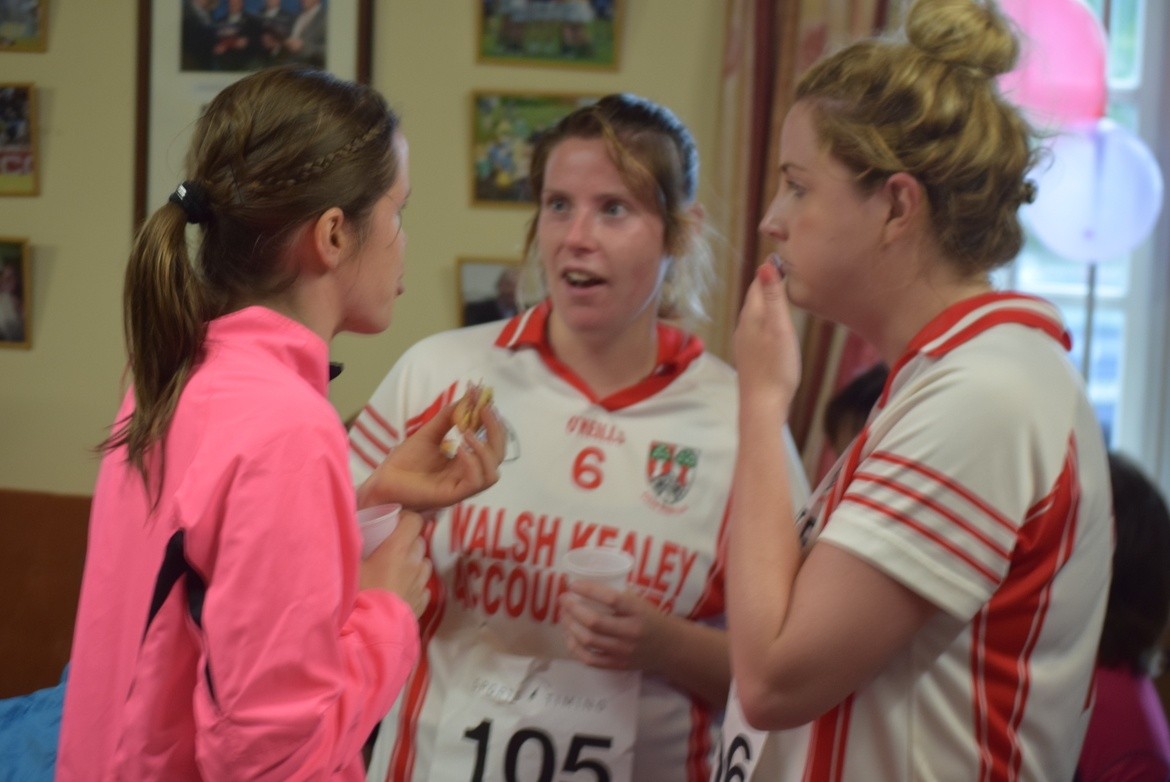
[704,0,1117,781]
[819,361,892,460]
[180,0,329,71]
[345,84,815,782]
[1077,451,1168,781]
[50,66,509,782]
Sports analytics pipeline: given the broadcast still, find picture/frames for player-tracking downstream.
[0,2,627,356]
